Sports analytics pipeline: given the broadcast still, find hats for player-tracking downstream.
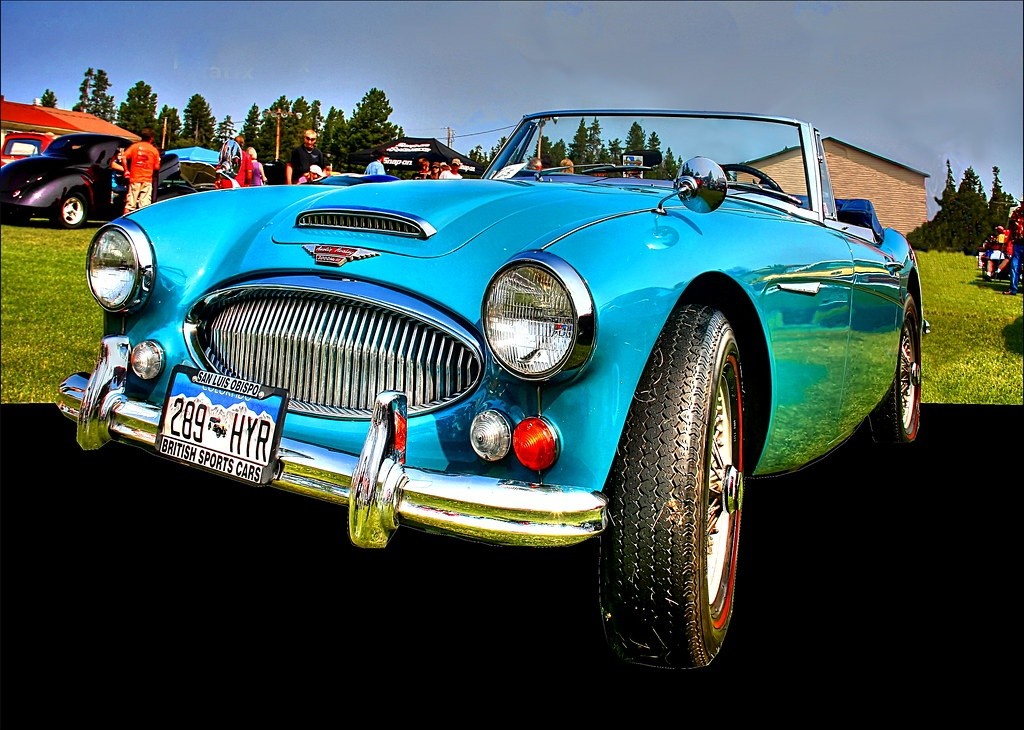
[995,225,1005,233]
[452,158,460,165]
[440,162,447,167]
[432,162,440,168]
[310,164,326,177]
[1019,195,1024,202]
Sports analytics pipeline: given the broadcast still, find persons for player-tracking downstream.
[980,194,1024,295]
[109,144,128,204]
[121,129,160,215]
[559,157,574,173]
[285,129,327,185]
[411,157,463,180]
[528,156,543,170]
[216,135,254,189]
[364,150,386,175]
[245,146,267,186]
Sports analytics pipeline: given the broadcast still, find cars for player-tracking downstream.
[0,132,403,229]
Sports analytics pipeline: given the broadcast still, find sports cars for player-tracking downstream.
[54,109,931,673]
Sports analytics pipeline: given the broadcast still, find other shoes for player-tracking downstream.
[1003,290,1015,295]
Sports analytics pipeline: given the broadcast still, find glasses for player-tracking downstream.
[305,136,315,142]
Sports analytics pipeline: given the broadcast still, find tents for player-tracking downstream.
[348,136,485,175]
